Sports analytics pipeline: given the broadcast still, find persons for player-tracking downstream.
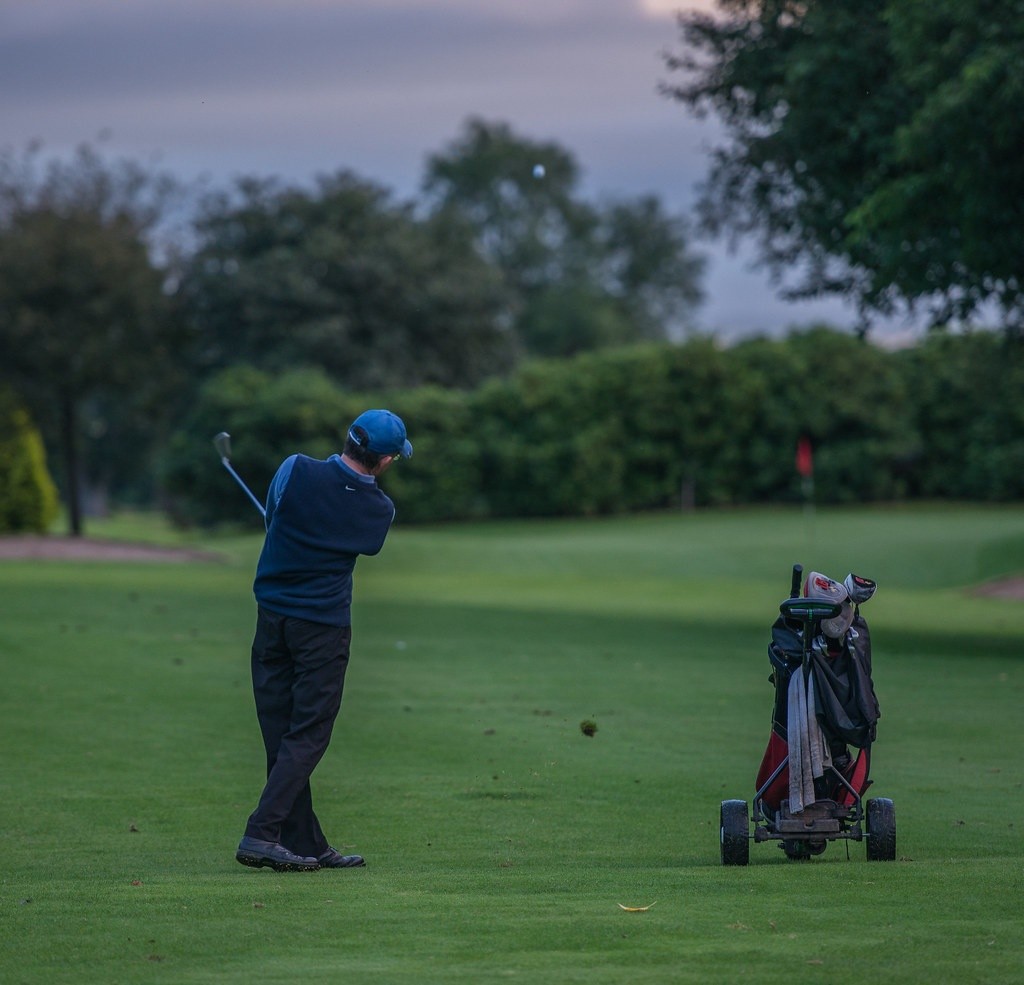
[235,408,413,872]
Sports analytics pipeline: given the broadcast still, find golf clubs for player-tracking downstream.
[791,563,803,600]
[211,431,270,518]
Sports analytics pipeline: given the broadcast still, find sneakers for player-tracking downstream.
[315,846,366,868]
[236,835,321,874]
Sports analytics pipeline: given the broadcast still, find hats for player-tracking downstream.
[348,409,412,460]
